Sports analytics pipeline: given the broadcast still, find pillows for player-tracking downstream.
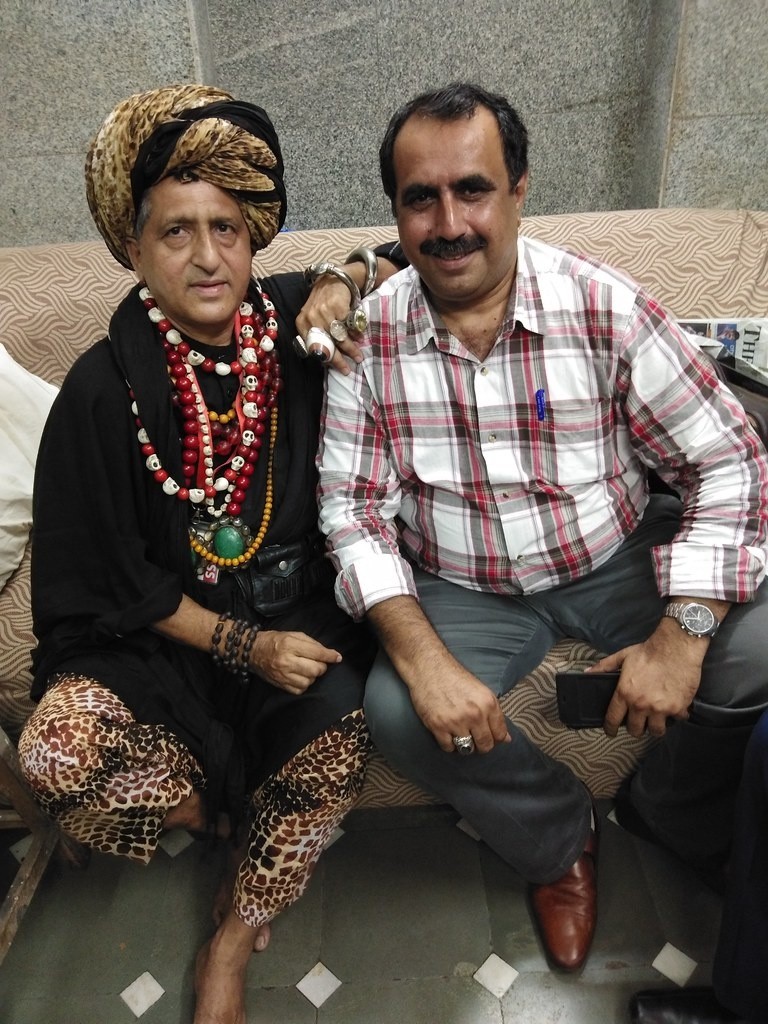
[0,342,60,591]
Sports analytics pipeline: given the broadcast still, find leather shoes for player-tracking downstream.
[630,986,742,1024]
[527,780,600,976]
[614,770,745,901]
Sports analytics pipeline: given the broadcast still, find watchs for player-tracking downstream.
[664,602,720,638]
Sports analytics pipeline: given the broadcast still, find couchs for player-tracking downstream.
[0,206,767,813]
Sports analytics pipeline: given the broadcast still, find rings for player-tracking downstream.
[345,311,367,332]
[453,735,474,756]
[306,327,335,363]
[330,320,348,342]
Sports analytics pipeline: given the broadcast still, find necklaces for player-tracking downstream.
[108,274,282,585]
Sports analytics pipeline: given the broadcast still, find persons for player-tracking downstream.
[15,83,410,1024]
[314,83,768,971]
[628,710,768,1024]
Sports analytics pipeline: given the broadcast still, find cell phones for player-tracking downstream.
[556,671,629,729]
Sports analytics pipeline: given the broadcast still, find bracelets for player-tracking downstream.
[211,611,259,685]
[303,263,361,311]
[345,246,378,297]
